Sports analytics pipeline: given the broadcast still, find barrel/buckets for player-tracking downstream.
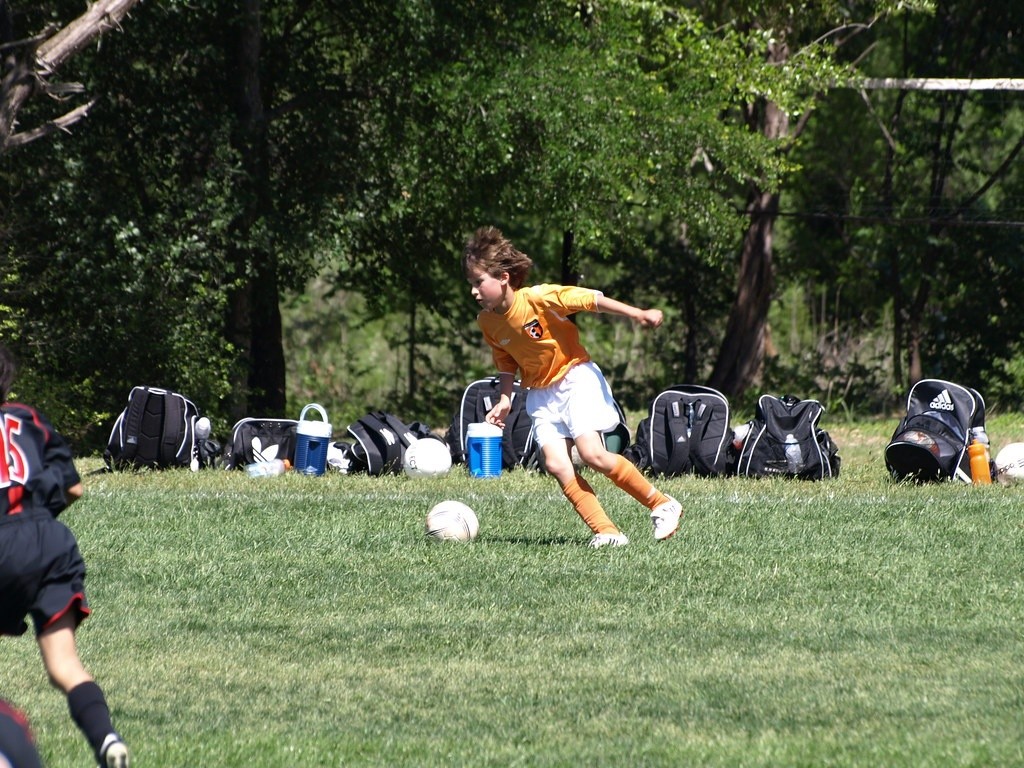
[466,421,504,478]
[293,402,332,478]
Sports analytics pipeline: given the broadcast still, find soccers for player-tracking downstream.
[403,437,452,479]
[424,500,480,542]
[995,442,1024,482]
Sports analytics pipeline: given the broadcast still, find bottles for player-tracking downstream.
[195,417,211,440]
[244,459,290,480]
[968,439,991,484]
[971,425,991,464]
[785,434,804,473]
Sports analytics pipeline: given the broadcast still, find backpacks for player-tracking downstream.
[739,393,842,480]
[445,376,544,473]
[635,383,738,477]
[102,386,200,473]
[347,412,418,478]
[885,379,996,483]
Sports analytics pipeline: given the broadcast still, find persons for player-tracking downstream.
[0,356,130,768]
[466,227,683,548]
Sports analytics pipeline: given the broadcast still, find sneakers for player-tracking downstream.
[587,533,629,550]
[650,495,683,540]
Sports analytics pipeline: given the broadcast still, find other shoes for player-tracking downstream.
[96,732,131,768]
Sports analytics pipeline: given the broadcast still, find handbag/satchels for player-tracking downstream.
[223,417,301,471]
